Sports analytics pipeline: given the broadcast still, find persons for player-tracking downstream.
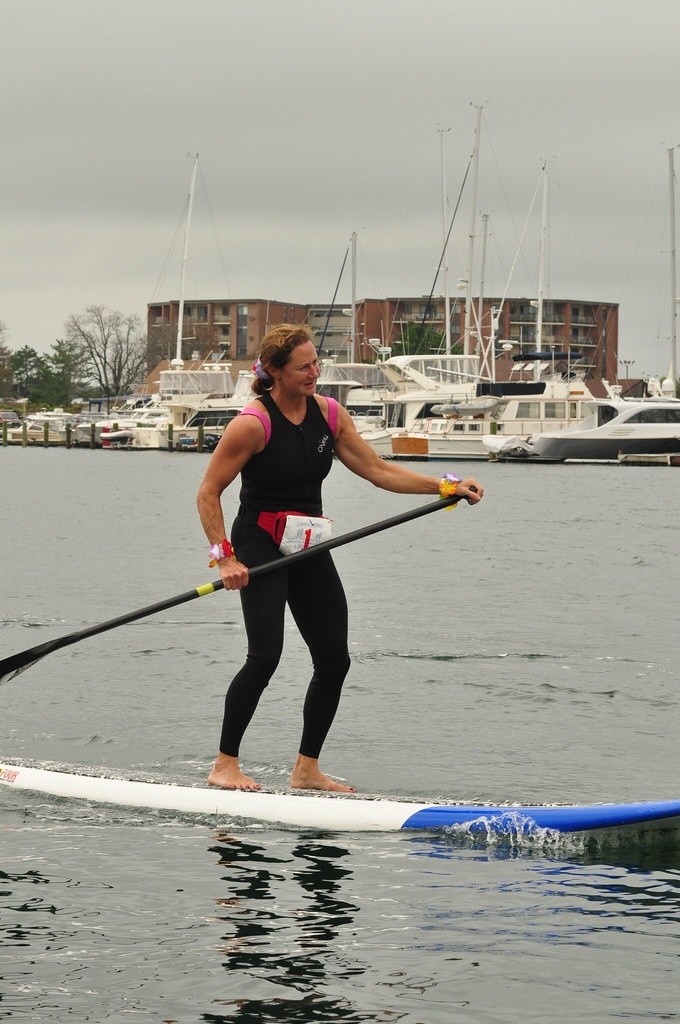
[197,322,484,790]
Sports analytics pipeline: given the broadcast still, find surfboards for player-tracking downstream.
[0,762,676,842]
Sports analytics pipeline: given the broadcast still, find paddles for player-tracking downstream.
[2,487,479,677]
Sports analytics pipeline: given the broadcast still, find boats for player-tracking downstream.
[0,101,679,469]
